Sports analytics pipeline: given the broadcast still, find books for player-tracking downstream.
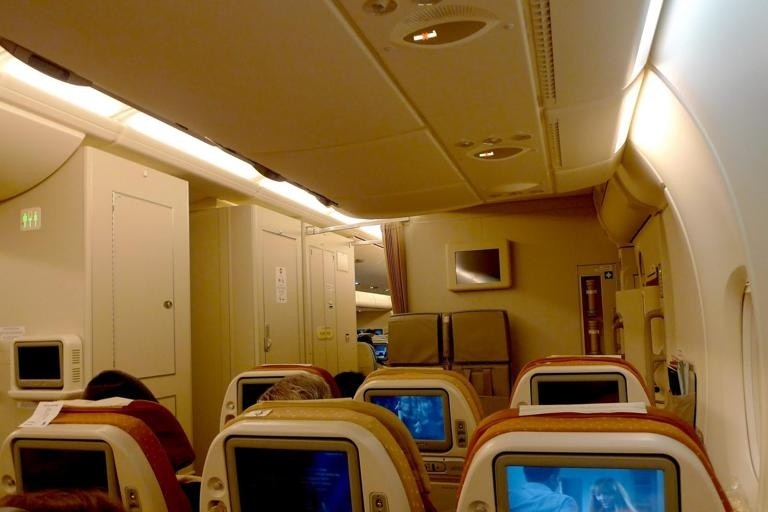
[666,353,690,396]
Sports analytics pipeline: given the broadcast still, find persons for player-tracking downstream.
[335,371,364,399]
[358,334,384,365]
[507,465,581,512]
[588,476,638,511]
[255,373,332,401]
[80,368,204,484]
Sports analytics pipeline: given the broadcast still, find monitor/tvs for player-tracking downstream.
[225,436,364,512]
[495,453,680,512]
[363,390,452,453]
[372,343,387,360]
[14,342,64,388]
[455,248,501,285]
[13,439,120,502]
[236,376,287,413]
[531,374,627,405]
